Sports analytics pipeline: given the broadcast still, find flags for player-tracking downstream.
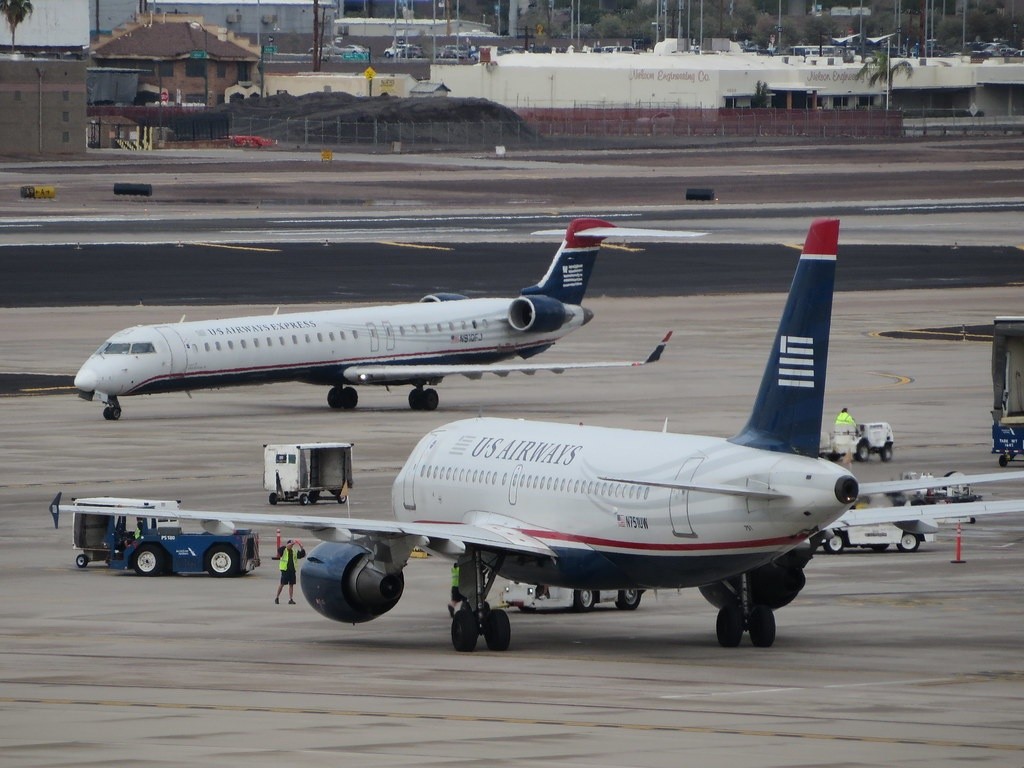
[340,480,350,499]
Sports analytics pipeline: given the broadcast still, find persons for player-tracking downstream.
[125,517,144,547]
[445,560,468,618]
[275,539,306,606]
[836,407,856,427]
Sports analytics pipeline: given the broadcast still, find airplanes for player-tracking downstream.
[48,217,1023,653]
[69,218,715,420]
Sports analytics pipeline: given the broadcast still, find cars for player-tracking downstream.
[384,44,411,58]
[307,44,333,55]
[398,46,427,59]
[966,41,1024,58]
[434,42,526,61]
[333,44,369,56]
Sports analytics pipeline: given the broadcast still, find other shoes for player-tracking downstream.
[448,605,455,617]
[275,598,279,604]
[288,599,296,604]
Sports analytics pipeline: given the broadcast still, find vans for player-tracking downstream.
[600,46,635,55]
